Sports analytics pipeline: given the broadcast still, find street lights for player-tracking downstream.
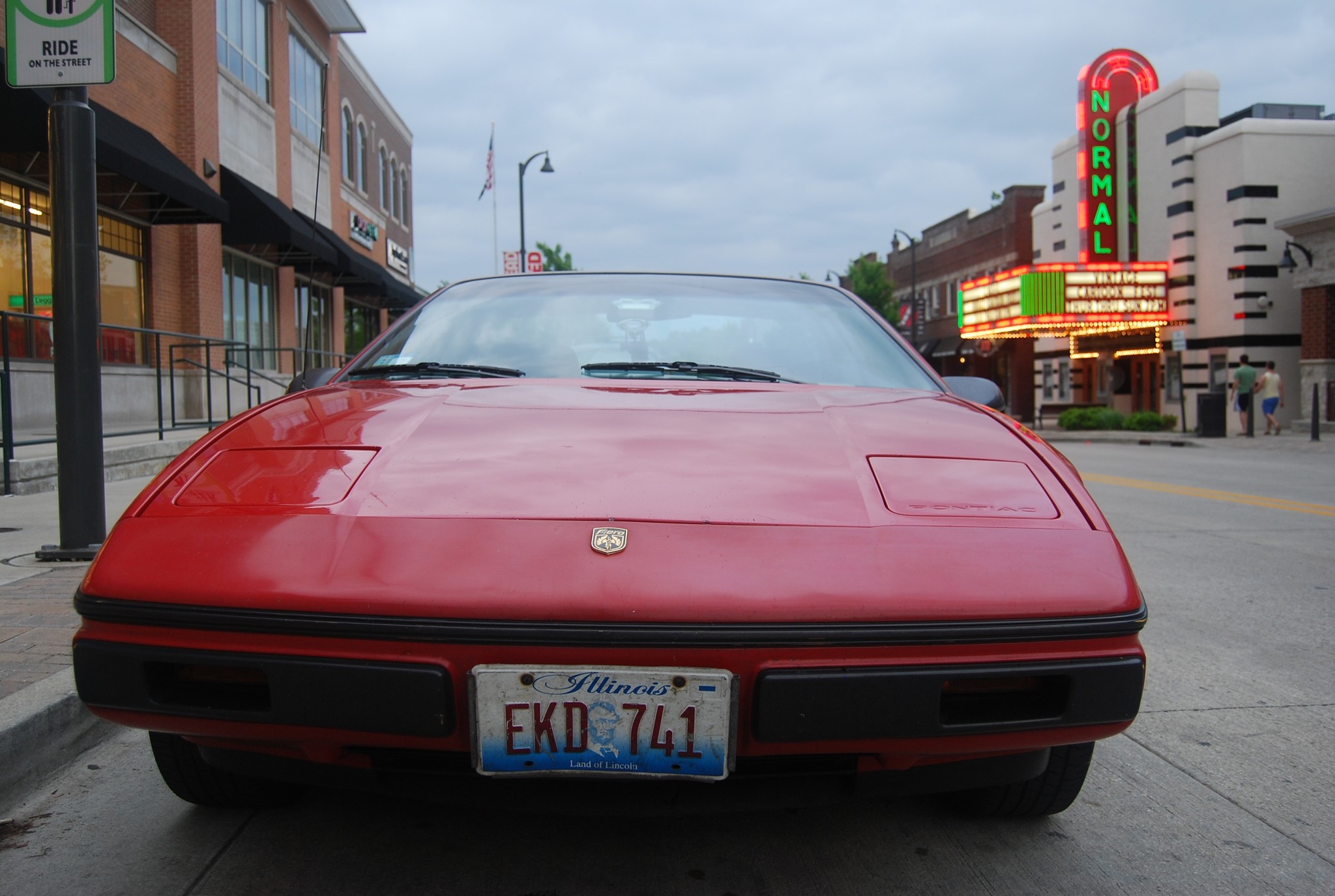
[517,148,557,271]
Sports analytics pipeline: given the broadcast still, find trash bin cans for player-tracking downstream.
[1197,392,1226,438]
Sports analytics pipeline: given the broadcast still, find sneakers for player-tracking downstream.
[1264,430,1270,434]
[1274,424,1281,436]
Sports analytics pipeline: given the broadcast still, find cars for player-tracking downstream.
[75,267,1150,819]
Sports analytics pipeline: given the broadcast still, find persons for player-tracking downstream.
[1253,360,1284,435]
[1230,354,1257,436]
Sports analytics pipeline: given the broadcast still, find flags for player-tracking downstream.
[477,127,494,199]
